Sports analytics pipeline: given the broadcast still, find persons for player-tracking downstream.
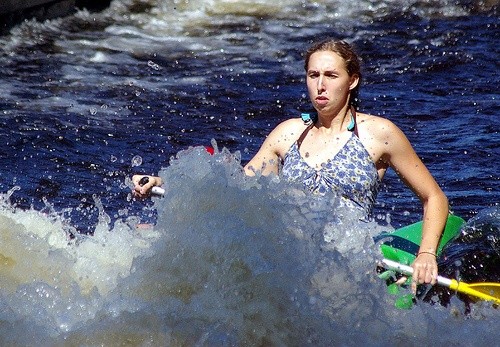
[132,37,448,296]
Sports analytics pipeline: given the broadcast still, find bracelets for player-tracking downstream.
[416,252,438,259]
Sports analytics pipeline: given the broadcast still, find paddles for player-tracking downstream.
[139,177,500,306]
